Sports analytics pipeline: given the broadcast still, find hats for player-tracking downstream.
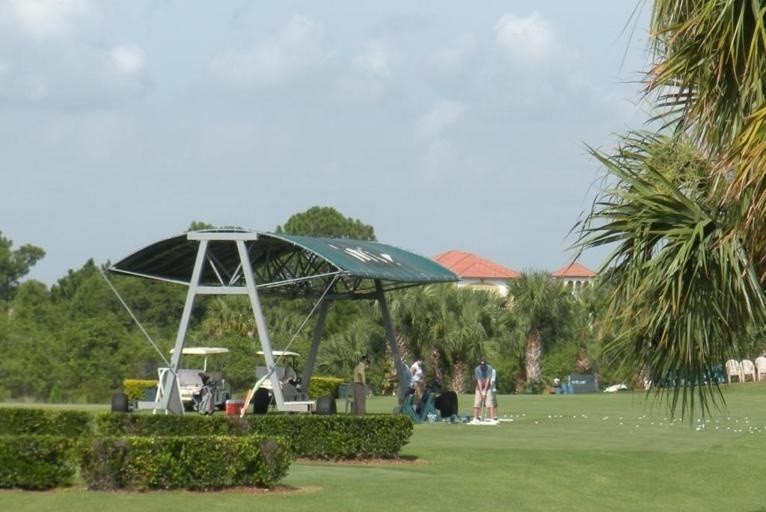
[359,356,370,364]
[479,359,487,365]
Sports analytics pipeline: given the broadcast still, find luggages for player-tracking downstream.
[198,385,214,415]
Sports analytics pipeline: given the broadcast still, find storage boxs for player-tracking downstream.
[225,400,246,414]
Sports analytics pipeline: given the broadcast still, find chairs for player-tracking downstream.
[344,389,355,414]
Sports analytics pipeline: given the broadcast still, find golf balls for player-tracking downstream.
[547,414,563,418]
[535,421,538,424]
[619,415,765,434]
[603,416,608,420]
[572,415,576,419]
[581,414,588,419]
[503,413,525,418]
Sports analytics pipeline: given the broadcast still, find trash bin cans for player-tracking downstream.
[254,388,268,414]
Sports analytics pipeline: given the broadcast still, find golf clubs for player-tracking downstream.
[481,398,489,421]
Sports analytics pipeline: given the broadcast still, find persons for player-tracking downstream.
[289,360,304,392]
[409,355,424,398]
[252,379,272,413]
[352,355,370,414]
[475,361,498,420]
[473,359,493,422]
[281,361,297,384]
[549,377,564,395]
